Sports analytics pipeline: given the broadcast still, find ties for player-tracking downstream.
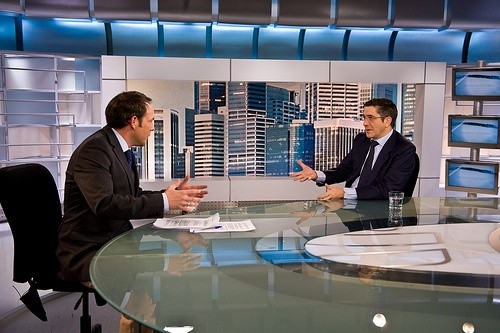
[126,150,133,174]
[357,141,379,188]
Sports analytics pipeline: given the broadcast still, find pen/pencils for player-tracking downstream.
[202,226,222,230]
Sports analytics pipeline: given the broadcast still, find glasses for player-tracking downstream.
[363,115,381,121]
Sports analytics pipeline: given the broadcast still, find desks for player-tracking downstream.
[89,196,500,333]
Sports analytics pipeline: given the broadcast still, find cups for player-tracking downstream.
[389,191,404,210]
[388,210,402,226]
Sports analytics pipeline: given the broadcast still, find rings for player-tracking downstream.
[186,201,190,207]
[188,256,191,260]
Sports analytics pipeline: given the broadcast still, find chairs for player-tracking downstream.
[0,163,103,333]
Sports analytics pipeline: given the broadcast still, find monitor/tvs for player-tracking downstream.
[452,67,500,101]
[444,159,499,195]
[448,114,500,149]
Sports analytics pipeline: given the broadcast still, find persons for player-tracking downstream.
[289,98,421,201]
[288,197,417,288]
[90,223,210,327]
[51,90,209,333]
